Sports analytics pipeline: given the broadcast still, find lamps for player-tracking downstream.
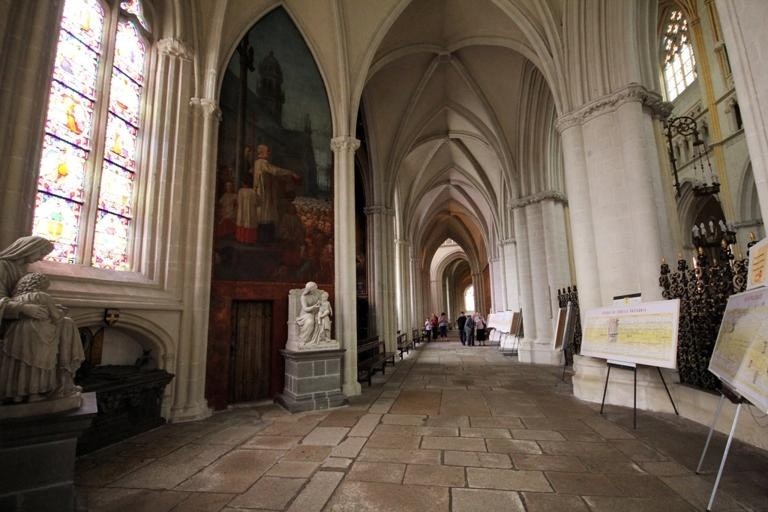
[667,115,720,197]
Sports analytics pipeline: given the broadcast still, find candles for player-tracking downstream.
[661,232,758,277]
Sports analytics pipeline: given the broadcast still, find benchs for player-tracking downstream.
[357,328,427,386]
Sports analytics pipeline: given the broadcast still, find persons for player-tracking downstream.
[0,235,87,401]
[429,311,439,342]
[298,282,333,346]
[424,317,432,343]
[438,312,449,342]
[457,311,467,345]
[215,139,333,278]
[315,291,334,324]
[474,312,487,346]
[463,314,476,346]
[2,272,68,404]
